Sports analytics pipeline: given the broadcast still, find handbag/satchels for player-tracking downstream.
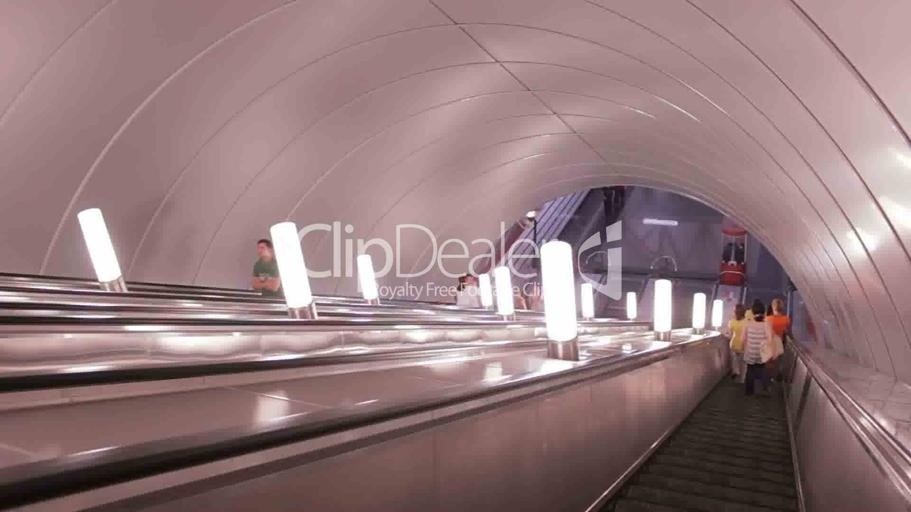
[759,321,784,363]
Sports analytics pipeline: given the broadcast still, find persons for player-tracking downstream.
[454,275,479,304]
[513,286,528,310]
[722,242,737,263]
[737,243,744,265]
[252,238,285,300]
[728,298,794,398]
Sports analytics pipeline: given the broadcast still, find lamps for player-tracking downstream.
[539,240,578,361]
[477,267,515,322]
[268,220,317,321]
[77,206,126,293]
[581,278,724,341]
[357,254,380,304]
